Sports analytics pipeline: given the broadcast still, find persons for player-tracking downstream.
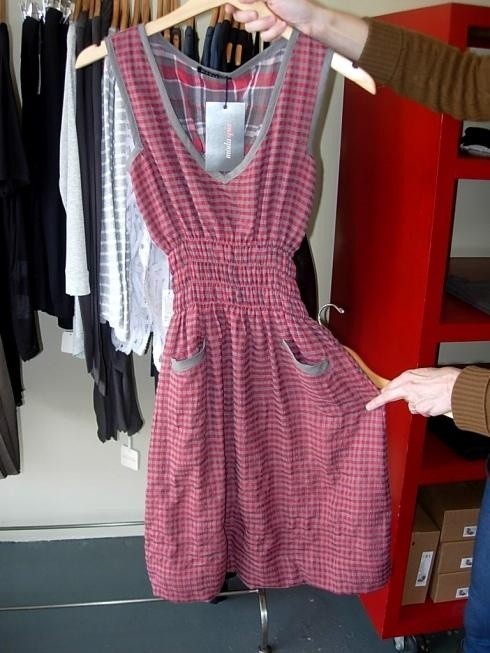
[223,2,490,653]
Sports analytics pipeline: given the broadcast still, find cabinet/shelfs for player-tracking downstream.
[328,2,490,643]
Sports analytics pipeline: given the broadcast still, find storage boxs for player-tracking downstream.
[434,569,473,605]
[439,501,482,543]
[438,537,478,574]
[402,506,441,606]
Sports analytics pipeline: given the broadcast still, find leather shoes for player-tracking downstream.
[413,631,464,652]
